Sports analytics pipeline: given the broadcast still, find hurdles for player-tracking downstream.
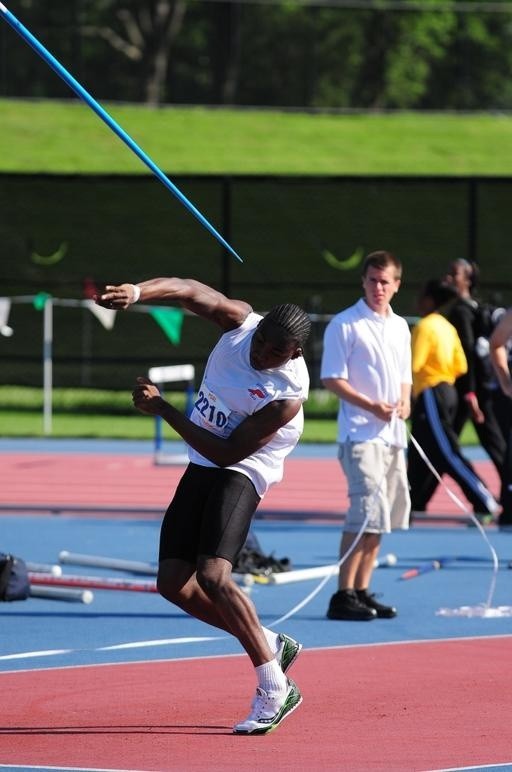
[148,363,197,464]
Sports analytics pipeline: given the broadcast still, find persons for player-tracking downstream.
[92,276,311,736]
[408,250,512,533]
[319,250,414,620]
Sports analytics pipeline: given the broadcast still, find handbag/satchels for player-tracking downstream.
[0,552,31,603]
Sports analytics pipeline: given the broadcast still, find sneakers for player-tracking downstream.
[232,677,303,736]
[361,593,397,619]
[274,631,304,675]
[326,591,378,621]
[468,504,504,527]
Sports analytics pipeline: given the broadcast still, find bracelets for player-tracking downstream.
[131,283,141,304]
